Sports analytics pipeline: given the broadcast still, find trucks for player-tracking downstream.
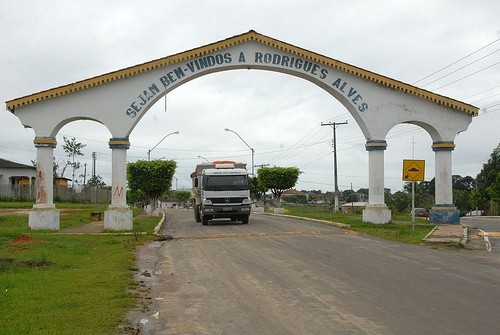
[190,160,258,225]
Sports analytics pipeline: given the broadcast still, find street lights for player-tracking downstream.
[224,127,255,178]
[147,130,180,161]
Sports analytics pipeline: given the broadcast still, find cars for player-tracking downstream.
[411,208,429,217]
